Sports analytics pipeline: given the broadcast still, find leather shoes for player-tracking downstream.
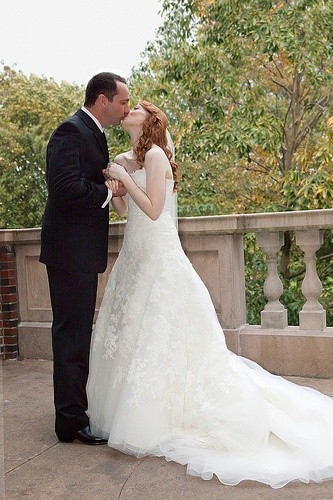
[63,425,109,445]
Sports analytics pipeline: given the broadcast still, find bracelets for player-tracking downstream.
[126,186,138,193]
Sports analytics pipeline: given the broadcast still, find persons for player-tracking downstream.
[39,71,133,443]
[85,101,228,490]
